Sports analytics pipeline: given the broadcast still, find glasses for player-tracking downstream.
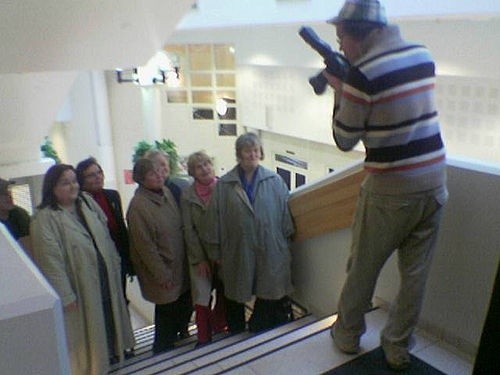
[336,30,347,45]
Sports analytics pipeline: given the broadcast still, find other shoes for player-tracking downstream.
[331,322,359,355]
[381,337,411,370]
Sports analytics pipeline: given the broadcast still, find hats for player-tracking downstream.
[326,0,387,25]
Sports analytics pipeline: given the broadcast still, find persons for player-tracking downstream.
[143,148,196,341]
[181,150,230,351]
[126,157,184,354]
[0,177,34,250]
[202,133,297,337]
[322,0,451,370]
[29,162,136,375]
[73,157,136,306]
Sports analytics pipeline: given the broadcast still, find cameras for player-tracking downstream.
[299,25,350,95]
